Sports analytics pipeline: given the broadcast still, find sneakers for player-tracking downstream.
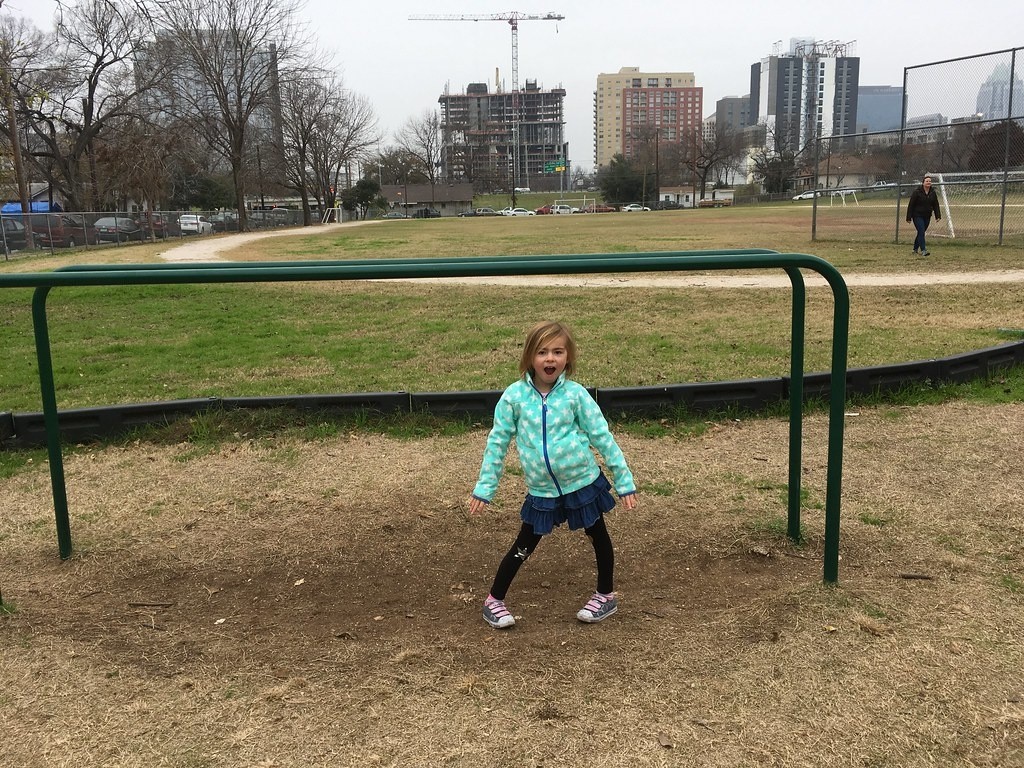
[482,599,515,629]
[576,594,618,623]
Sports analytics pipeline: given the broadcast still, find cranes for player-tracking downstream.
[405,10,567,193]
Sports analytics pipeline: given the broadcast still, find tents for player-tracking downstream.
[1,198,64,225]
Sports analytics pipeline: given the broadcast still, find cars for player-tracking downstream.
[498,207,514,216]
[382,212,412,219]
[506,208,536,216]
[134,212,183,239]
[828,185,858,196]
[93,216,147,242]
[458,209,477,218]
[697,198,732,208]
[655,200,685,211]
[620,204,651,213]
[175,214,212,235]
[548,204,616,213]
[412,207,443,218]
[534,203,552,215]
[0,217,43,255]
[208,214,237,232]
[230,209,322,228]
[475,208,501,216]
[23,213,102,248]
[792,189,822,201]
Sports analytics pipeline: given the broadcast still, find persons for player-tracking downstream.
[905,176,942,256]
[467,320,638,630]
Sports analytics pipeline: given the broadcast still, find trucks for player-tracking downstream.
[865,179,898,192]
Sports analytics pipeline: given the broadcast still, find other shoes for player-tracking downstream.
[913,250,920,255]
[921,250,930,256]
